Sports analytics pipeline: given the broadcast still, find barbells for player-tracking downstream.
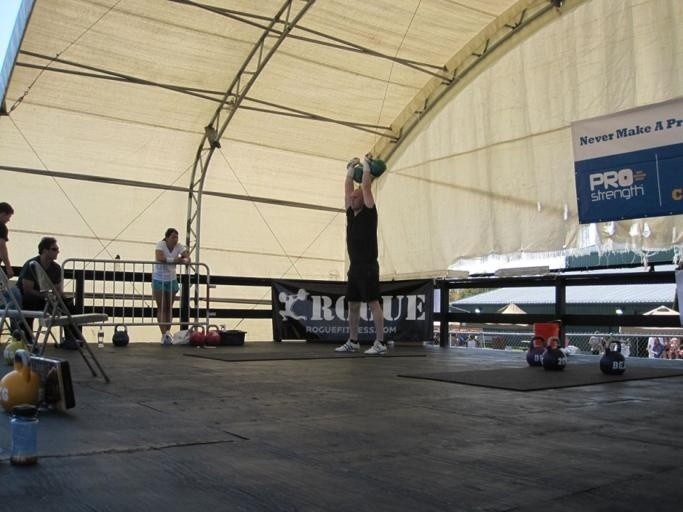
[278,288,305,303]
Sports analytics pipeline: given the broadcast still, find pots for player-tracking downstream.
[215,329,247,346]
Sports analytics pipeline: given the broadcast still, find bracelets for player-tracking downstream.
[363,163,370,172]
[347,167,355,177]
[167,257,174,263]
[184,258,189,263]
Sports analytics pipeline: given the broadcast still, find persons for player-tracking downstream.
[0,202,14,278]
[423,328,478,349]
[589,330,683,359]
[334,153,389,354]
[151,228,191,345]
[0,255,23,341]
[16,237,81,350]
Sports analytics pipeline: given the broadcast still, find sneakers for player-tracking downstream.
[364,340,387,354]
[334,340,360,352]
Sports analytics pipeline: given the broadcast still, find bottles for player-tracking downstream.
[8,404,39,468]
[97,331,104,349]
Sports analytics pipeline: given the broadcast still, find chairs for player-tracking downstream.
[29,260,110,382]
[0,266,58,351]
